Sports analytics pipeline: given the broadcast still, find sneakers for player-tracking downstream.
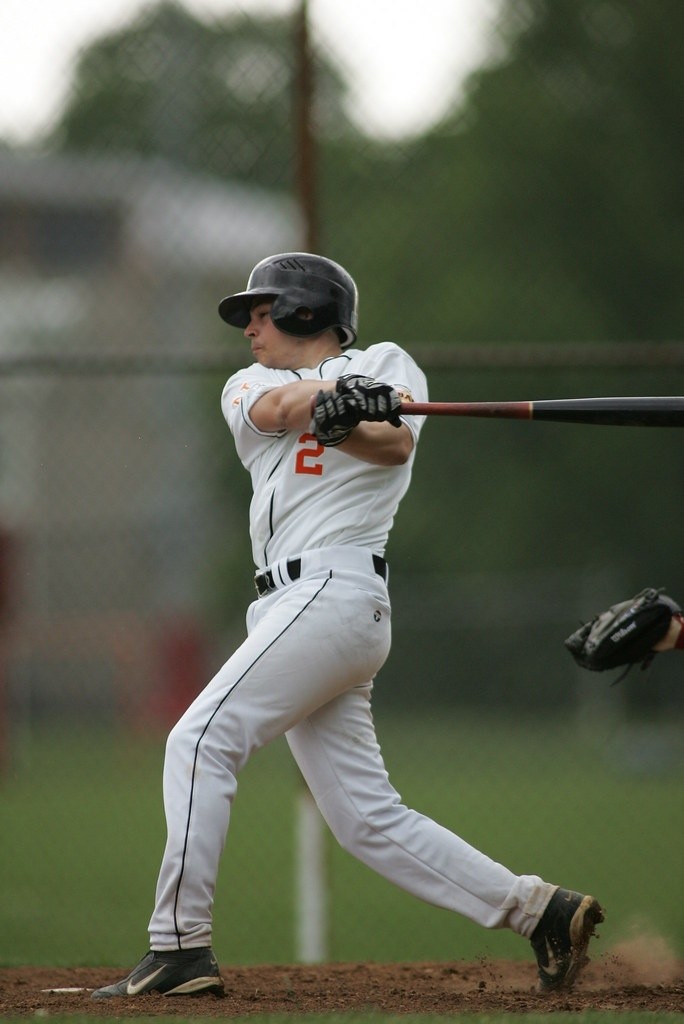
[529,887,604,991]
[91,948,225,999]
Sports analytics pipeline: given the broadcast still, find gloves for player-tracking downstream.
[336,374,402,428]
[312,389,360,447]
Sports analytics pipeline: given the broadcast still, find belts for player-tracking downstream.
[254,554,387,597]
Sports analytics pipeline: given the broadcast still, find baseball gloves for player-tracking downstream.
[566,581,683,688]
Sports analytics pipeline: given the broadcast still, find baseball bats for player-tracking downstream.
[308,394,684,426]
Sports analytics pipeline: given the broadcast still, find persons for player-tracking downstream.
[562,586,684,671]
[93,251,605,1001]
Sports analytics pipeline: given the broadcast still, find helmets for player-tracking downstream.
[217,253,359,351]
